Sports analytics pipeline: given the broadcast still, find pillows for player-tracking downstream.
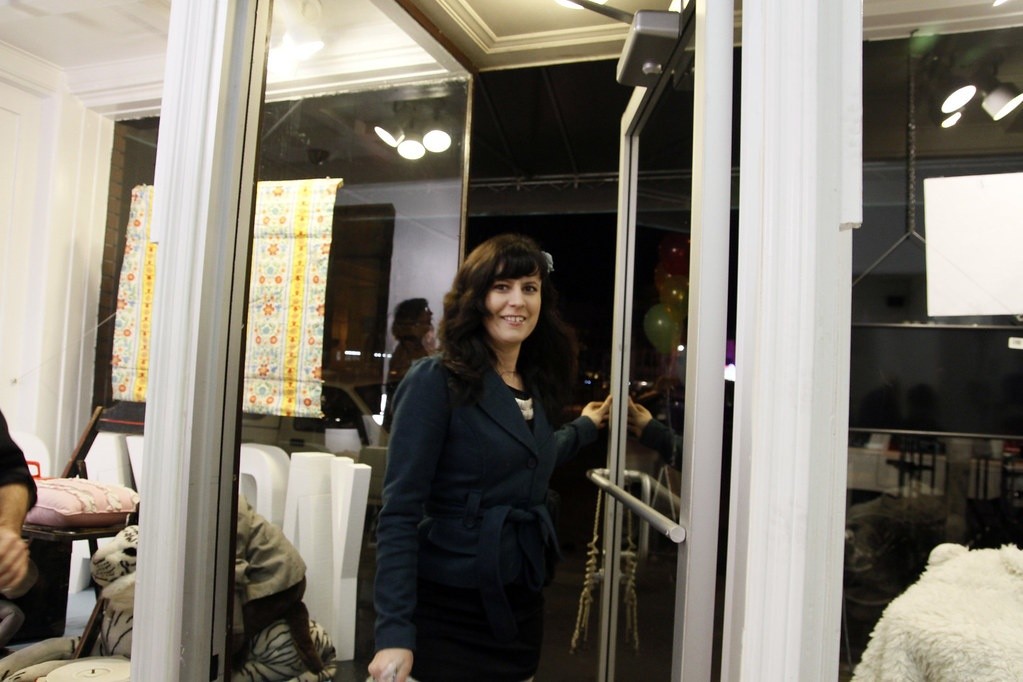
[25,477,140,527]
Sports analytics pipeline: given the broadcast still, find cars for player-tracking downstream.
[97,364,402,511]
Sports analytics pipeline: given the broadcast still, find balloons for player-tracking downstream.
[645,236,689,358]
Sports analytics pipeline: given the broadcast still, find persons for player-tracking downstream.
[627,396,683,472]
[384,298,432,427]
[368,235,613,682]
[0,409,38,595]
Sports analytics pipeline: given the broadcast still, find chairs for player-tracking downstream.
[276,440,389,551]
[843,513,916,673]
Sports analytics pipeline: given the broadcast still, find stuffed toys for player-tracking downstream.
[849,543,1023,682]
[0,519,336,682]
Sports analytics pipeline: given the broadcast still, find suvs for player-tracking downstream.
[845,319,1023,622]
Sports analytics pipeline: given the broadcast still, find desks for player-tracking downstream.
[19,522,131,661]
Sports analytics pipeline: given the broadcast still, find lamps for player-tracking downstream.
[922,51,1023,129]
[374,109,453,160]
